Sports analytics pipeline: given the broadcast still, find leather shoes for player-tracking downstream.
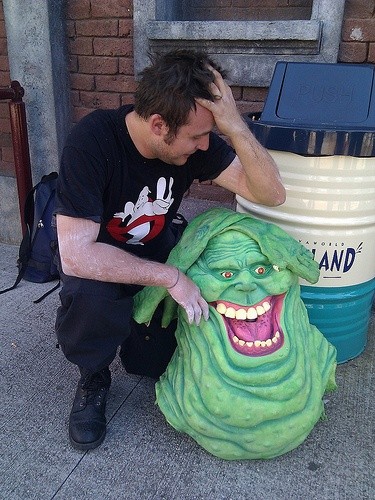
[69,366,111,450]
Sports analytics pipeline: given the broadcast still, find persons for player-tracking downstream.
[54,47,287,451]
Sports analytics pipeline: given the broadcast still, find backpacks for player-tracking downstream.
[17,171,61,283]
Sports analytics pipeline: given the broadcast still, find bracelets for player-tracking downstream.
[166,265,180,289]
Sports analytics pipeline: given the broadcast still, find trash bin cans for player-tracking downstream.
[234,62,375,368]
